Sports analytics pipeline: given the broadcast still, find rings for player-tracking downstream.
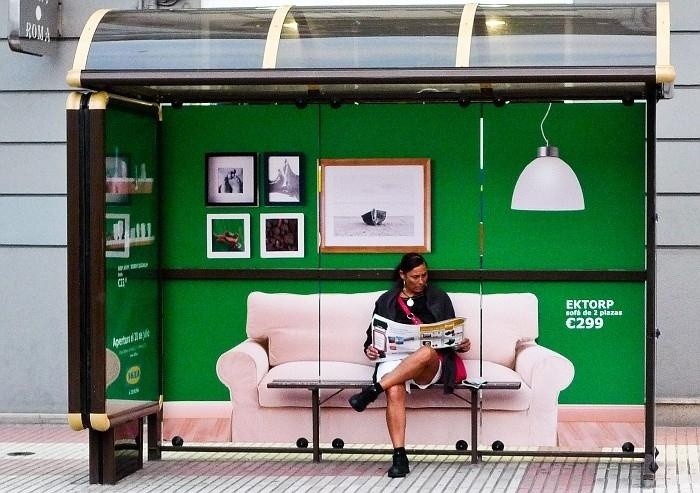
[368,350,371,352]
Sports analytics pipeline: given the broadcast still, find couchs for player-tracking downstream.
[216,285,580,448]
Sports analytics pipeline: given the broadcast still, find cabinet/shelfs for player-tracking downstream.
[104,175,156,250]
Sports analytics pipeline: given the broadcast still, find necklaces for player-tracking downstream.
[402,289,421,307]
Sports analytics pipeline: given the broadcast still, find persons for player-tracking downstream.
[348,251,472,477]
[281,157,298,197]
[275,167,284,189]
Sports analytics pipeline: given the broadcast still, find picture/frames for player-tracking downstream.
[204,148,259,208]
[257,210,306,260]
[205,211,253,259]
[261,149,306,208]
[310,157,435,254]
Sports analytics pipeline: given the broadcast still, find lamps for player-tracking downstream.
[507,101,588,214]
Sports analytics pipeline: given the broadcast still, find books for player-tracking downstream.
[370,312,468,361]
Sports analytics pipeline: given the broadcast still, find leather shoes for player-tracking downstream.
[388,455,410,478]
[349,385,377,412]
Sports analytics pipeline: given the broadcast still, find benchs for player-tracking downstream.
[263,376,525,466]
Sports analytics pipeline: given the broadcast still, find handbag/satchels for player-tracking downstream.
[440,353,467,384]
